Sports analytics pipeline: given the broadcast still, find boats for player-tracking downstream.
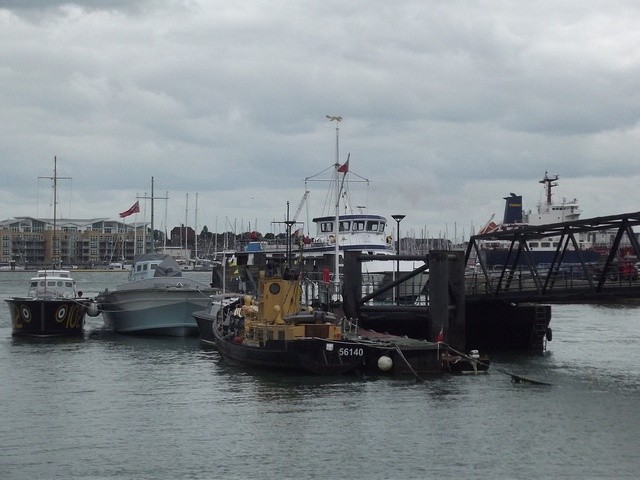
[4,156,93,337]
[95,251,214,336]
[191,215,258,348]
[213,201,448,373]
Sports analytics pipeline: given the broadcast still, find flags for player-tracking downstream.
[337,152,351,173]
[118,200,141,218]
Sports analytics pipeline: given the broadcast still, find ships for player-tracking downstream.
[476,171,603,281]
[212,116,428,307]
